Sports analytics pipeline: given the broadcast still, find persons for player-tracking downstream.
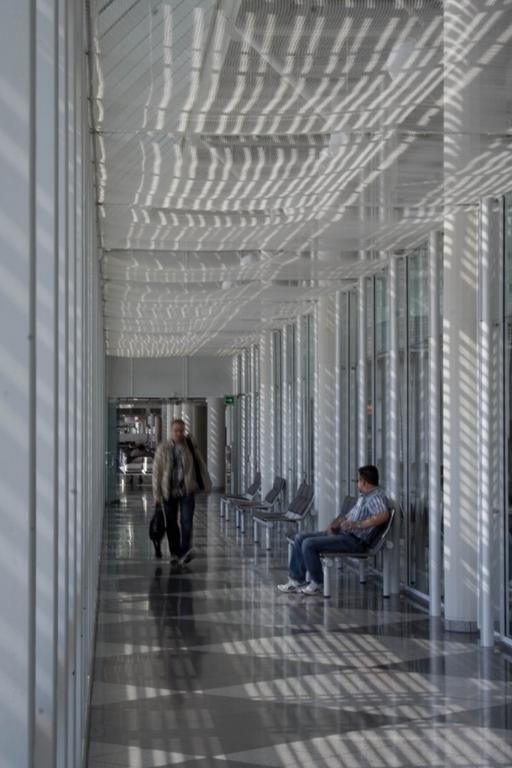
[152,419,212,565]
[276,465,390,597]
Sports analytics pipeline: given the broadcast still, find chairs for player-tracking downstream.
[234,476,286,533]
[286,494,393,598]
[220,472,261,521]
[252,483,315,550]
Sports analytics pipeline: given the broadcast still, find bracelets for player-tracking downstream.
[356,520,361,529]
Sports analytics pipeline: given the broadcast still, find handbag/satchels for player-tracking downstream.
[149,501,165,542]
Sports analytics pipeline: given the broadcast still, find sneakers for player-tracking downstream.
[172,554,191,567]
[277,582,321,595]
